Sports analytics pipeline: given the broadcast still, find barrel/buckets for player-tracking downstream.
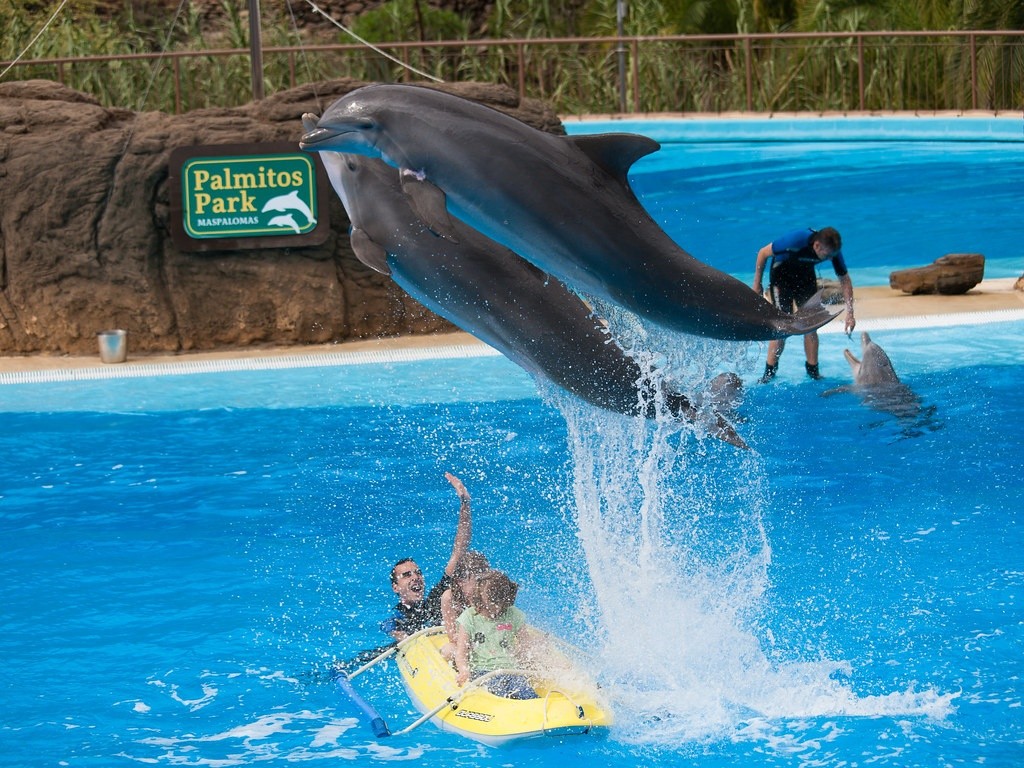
[98,329,127,363]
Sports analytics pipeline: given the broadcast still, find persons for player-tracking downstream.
[752,225,856,384]
[379,471,570,701]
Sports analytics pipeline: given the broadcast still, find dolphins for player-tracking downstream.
[297,82,846,453]
[819,332,947,444]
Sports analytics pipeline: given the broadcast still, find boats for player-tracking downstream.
[395,606,613,747]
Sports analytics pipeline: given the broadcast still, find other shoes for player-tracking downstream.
[805,361,821,380]
[758,362,778,385]
